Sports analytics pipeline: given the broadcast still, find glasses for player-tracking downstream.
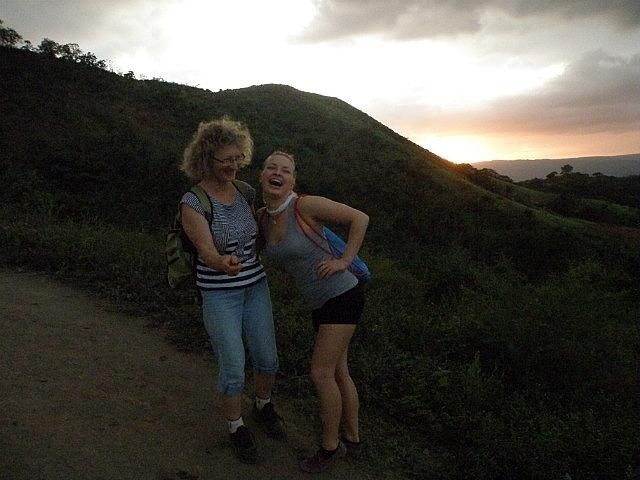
[213,153,245,164]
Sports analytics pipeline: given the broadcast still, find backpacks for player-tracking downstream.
[321,225,372,284]
[164,179,247,289]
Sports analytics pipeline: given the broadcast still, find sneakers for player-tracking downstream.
[250,401,288,441]
[300,433,362,473]
[228,422,261,465]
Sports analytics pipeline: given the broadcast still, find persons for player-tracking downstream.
[178,115,286,463]
[258,151,369,473]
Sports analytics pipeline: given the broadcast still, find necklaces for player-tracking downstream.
[265,191,297,224]
